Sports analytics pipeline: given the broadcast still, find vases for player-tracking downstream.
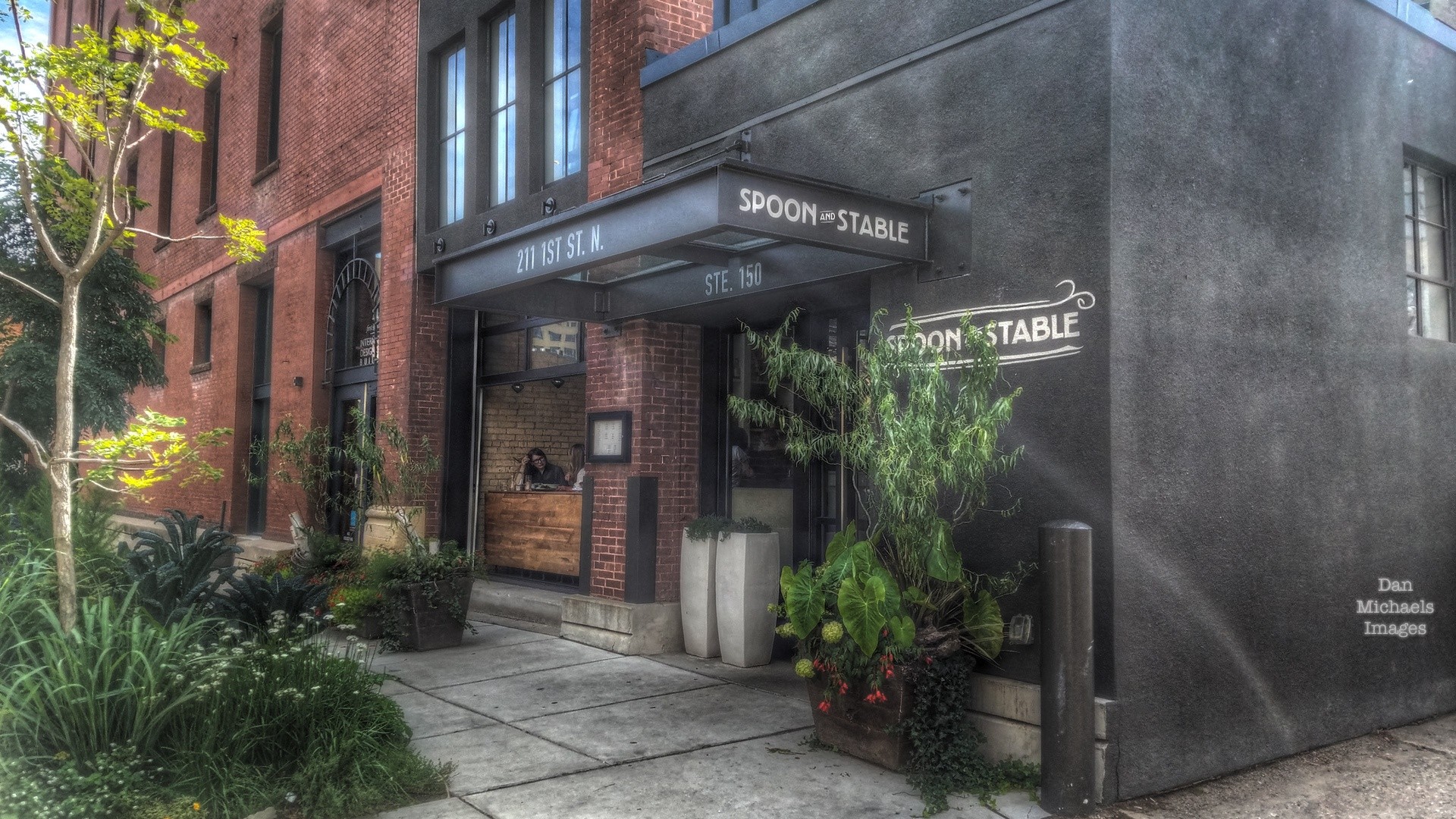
[807,664,912,772]
[353,617,384,639]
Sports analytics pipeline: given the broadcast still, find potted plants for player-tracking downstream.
[716,516,779,667]
[367,541,490,654]
[679,515,720,658]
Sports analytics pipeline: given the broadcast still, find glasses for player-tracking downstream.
[531,456,545,464]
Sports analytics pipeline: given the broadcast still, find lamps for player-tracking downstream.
[512,332,524,392]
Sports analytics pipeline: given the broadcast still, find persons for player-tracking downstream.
[556,443,585,491]
[516,448,569,486]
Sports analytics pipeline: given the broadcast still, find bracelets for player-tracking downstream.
[518,472,525,474]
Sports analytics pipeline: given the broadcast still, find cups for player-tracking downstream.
[525,475,532,492]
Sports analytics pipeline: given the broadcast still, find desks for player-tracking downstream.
[485,491,582,584]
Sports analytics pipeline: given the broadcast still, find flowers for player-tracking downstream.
[326,584,383,623]
[768,519,1004,716]
